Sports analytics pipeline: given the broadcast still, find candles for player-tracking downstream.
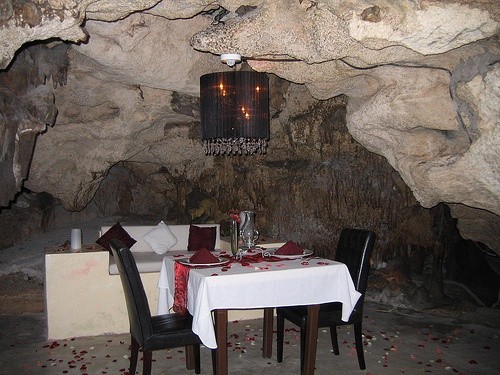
[71,229,81,250]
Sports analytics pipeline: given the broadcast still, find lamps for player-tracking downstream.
[221,54,242,66]
[239,211,259,247]
[200,71,269,155]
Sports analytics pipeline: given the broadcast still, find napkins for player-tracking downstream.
[190,248,220,264]
[275,240,304,255]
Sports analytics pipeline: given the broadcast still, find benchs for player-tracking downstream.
[99,224,220,274]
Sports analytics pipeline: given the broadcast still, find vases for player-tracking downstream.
[230,220,237,254]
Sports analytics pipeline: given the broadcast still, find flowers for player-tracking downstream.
[225,208,241,224]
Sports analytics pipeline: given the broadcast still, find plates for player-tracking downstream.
[270,250,313,259]
[178,258,230,266]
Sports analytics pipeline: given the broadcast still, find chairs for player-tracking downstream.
[277,227,375,370]
[109,238,216,375]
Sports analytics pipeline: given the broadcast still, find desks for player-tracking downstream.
[158,248,363,375]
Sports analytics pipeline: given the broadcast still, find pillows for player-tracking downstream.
[188,225,217,250]
[143,221,178,255]
[95,221,137,256]
